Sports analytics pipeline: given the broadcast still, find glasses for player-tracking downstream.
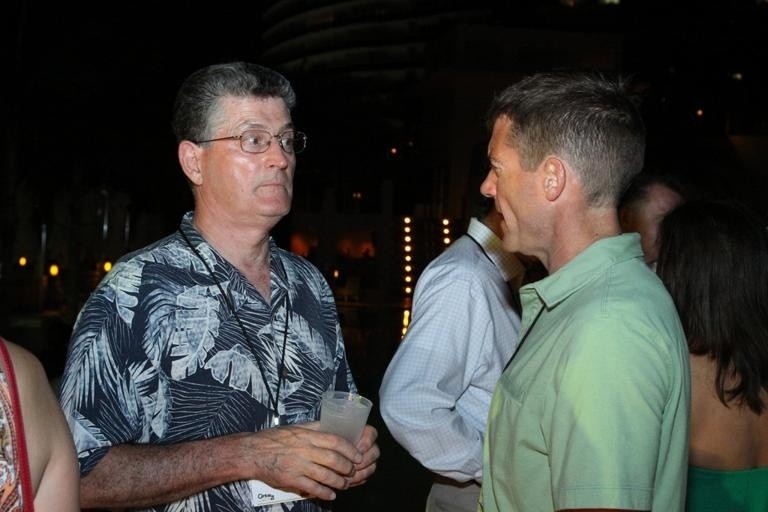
[196,129,308,154]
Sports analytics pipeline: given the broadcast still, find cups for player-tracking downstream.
[320,390,373,448]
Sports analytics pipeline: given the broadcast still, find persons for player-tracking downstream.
[648,181,767,512]
[58,61,382,512]
[0,337,82,512]
[614,173,688,273]
[376,121,540,511]
[473,64,693,512]
